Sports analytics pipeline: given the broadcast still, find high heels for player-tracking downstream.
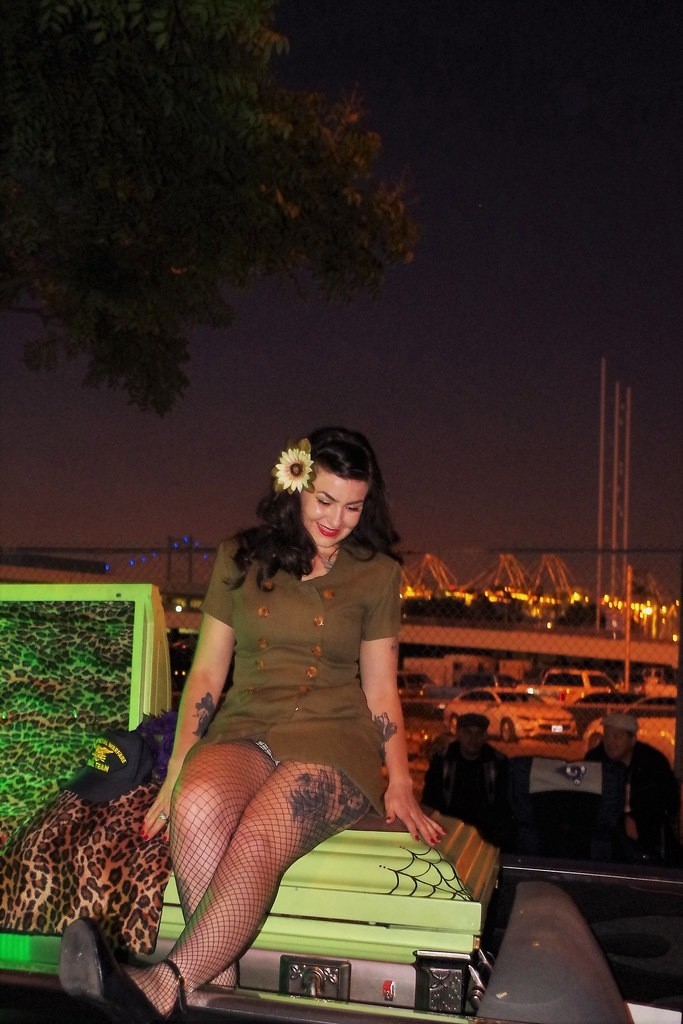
[58,917,187,1024]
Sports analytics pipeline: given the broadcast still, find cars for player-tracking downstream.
[444,685,579,742]
[581,697,676,770]
[541,670,617,704]
[400,698,453,757]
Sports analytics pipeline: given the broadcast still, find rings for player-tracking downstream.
[158,811,168,820]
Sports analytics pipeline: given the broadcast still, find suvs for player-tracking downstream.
[457,674,523,689]
[396,671,437,696]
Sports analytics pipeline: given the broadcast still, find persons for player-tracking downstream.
[584,714,674,866]
[59,424,447,1024]
[421,713,516,853]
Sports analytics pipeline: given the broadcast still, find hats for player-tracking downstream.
[600,714,637,733]
[457,713,489,728]
[60,729,154,801]
